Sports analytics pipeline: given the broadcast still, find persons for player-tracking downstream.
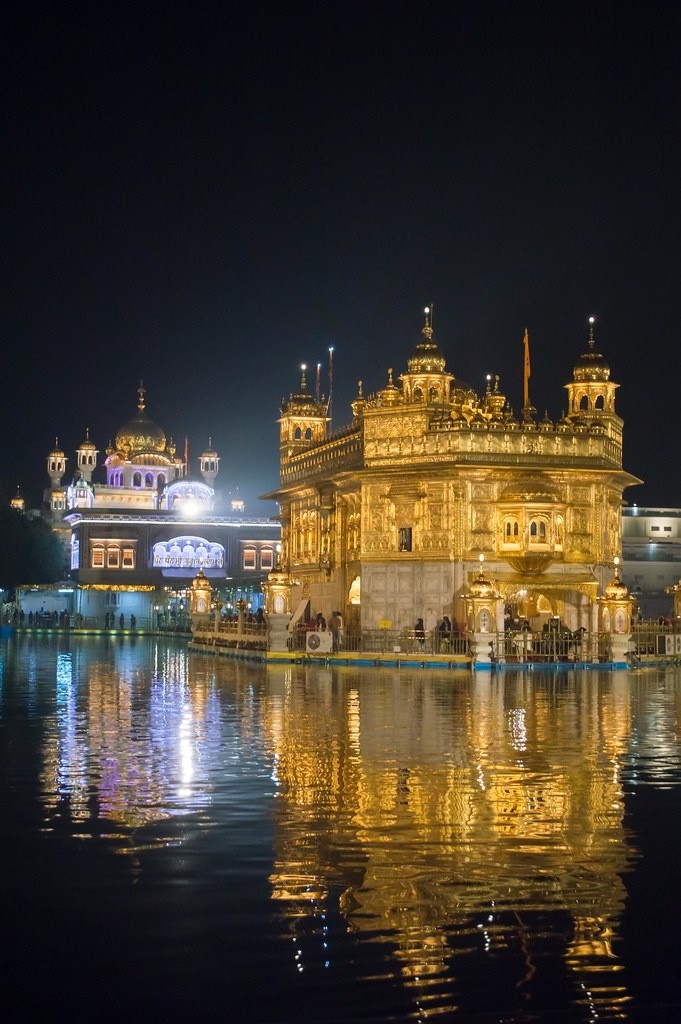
[105,612,109,629]
[511,619,522,630]
[316,613,326,633]
[110,612,115,629]
[439,616,451,638]
[522,622,531,631]
[130,614,136,629]
[415,618,425,651]
[327,611,343,650]
[120,613,124,629]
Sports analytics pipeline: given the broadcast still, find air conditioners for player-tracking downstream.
[305,631,333,654]
[656,634,681,655]
